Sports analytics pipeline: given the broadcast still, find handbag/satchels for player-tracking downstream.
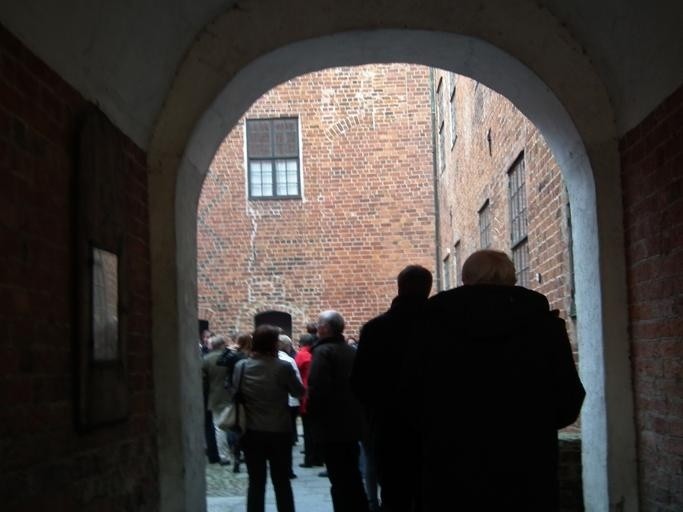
[218,363,247,442]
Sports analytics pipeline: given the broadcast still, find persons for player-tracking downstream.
[232,324,305,512]
[305,310,370,512]
[277,324,328,478]
[348,250,584,512]
[200,329,254,472]
